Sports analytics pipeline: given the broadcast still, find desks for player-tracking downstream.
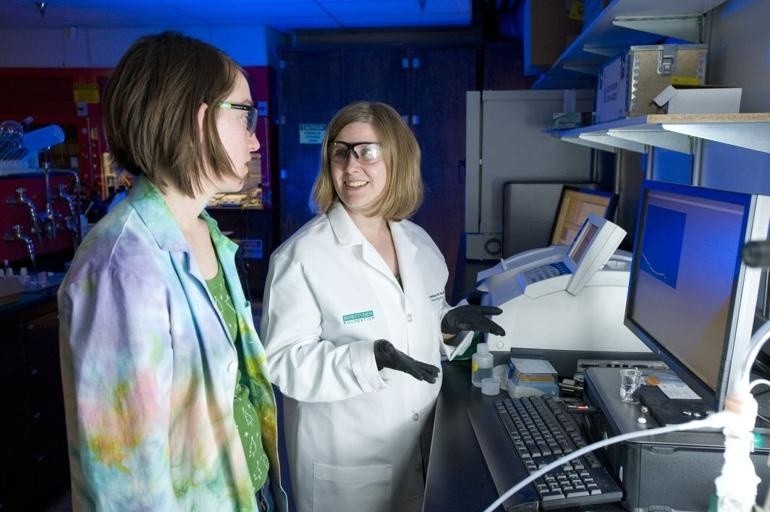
[419,352,672,511]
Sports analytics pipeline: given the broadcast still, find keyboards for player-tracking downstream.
[463,395,624,512]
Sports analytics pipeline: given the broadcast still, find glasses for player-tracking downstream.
[327,140,384,165]
[216,100,258,134]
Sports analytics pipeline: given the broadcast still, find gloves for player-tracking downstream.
[441,305,505,337]
[373,339,439,384]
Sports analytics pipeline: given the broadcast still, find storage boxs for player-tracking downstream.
[593,41,709,124]
[649,81,744,115]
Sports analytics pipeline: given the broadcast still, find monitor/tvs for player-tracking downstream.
[544,184,620,246]
[624,178,770,435]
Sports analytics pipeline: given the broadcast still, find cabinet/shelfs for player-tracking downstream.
[1,284,73,511]
[528,0,770,158]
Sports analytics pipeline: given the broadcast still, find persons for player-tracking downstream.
[57,31,289,512]
[260,100,505,512]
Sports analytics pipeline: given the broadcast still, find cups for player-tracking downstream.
[0,260,28,280]
[619,368,642,406]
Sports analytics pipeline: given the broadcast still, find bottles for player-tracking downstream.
[472,342,494,387]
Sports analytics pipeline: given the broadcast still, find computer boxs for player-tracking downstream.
[580,367,770,512]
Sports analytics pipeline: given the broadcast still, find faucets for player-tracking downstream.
[2,160,84,273]
